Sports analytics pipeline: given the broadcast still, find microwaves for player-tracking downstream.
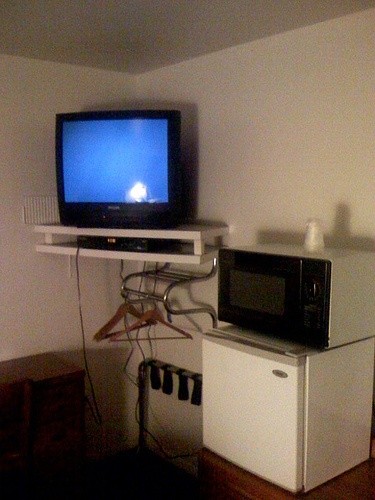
[216,241,374,350]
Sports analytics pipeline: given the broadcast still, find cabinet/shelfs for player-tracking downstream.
[198,450,375,500]
[0,352,86,474]
[34,222,229,265]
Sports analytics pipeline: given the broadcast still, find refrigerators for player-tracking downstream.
[201,324,375,493]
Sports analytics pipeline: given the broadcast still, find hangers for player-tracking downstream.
[109,296,193,340]
[93,289,157,345]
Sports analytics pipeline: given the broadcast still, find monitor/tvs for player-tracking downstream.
[56,110,182,231]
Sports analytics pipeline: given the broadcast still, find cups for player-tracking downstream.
[303,219,326,256]
